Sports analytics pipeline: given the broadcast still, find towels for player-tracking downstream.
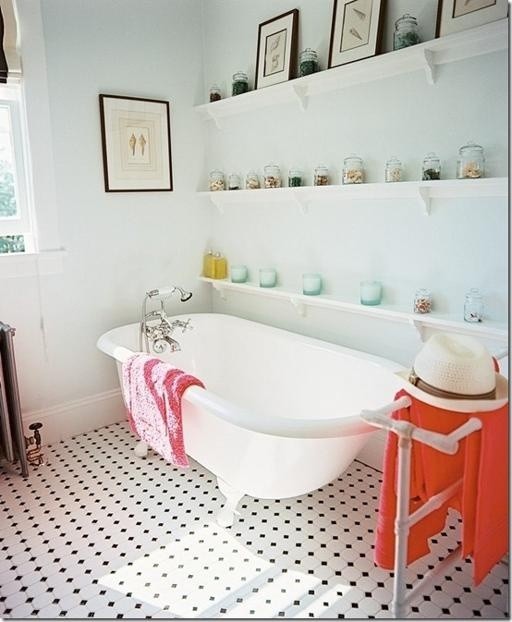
[371,356,509,588]
[116,349,209,471]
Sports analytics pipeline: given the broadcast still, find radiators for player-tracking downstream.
[0,321,44,479]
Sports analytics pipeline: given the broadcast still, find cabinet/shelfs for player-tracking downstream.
[194,17,509,340]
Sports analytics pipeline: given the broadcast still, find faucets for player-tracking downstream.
[162,335,182,354]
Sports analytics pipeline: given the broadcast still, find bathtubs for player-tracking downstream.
[95,312,412,528]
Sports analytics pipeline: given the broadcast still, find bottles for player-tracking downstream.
[314,163,329,185]
[456,133,487,180]
[207,11,418,102]
[341,152,365,184]
[288,166,303,187]
[422,150,442,180]
[207,159,283,192]
[413,288,434,315]
[463,286,486,324]
[203,249,217,277]
[384,156,403,182]
[212,253,228,280]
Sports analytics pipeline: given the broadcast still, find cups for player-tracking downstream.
[229,263,249,284]
[361,282,382,308]
[258,266,278,288]
[302,273,323,295]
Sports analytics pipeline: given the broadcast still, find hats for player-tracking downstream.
[395,335,508,412]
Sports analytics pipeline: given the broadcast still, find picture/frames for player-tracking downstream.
[325,0,389,69]
[95,92,175,194]
[252,6,300,91]
[433,0,510,41]
